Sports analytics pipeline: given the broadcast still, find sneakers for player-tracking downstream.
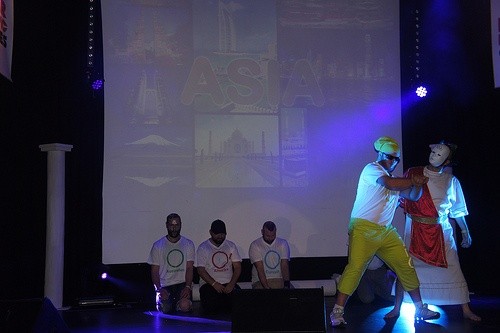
[330,309,347,326]
[414,305,440,321]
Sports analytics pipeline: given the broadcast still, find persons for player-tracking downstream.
[329,136,440,326]
[332,236,391,302]
[384,145,481,322]
[194,220,242,311]
[248,221,296,289]
[146,213,195,313]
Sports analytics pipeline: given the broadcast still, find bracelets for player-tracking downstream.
[212,281,217,286]
[284,280,291,287]
[187,286,192,290]
[462,229,468,234]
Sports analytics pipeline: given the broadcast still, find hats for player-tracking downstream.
[381,142,401,158]
[374,137,395,151]
[211,220,225,234]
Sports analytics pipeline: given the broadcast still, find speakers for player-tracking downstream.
[0,296,69,333]
[232,287,327,333]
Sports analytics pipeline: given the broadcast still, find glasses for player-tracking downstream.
[382,153,400,162]
